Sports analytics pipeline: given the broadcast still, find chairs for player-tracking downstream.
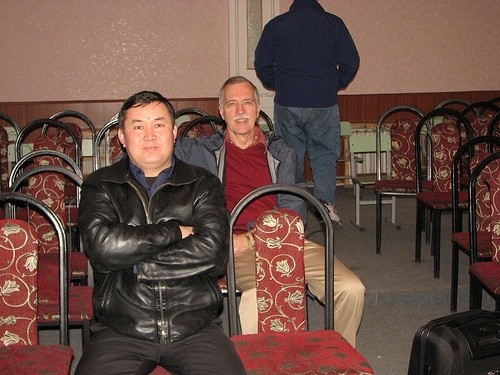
[374,100,500,312]
[349,131,400,231]
[225,184,375,375]
[174,106,273,138]
[0,111,122,375]
[337,121,364,199]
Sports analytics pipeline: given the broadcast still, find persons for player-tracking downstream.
[253,0,360,229]
[173,76,366,349]
[77,91,247,375]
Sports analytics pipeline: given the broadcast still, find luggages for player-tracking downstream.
[408,309,500,375]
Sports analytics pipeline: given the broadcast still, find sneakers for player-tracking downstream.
[314,201,343,227]
